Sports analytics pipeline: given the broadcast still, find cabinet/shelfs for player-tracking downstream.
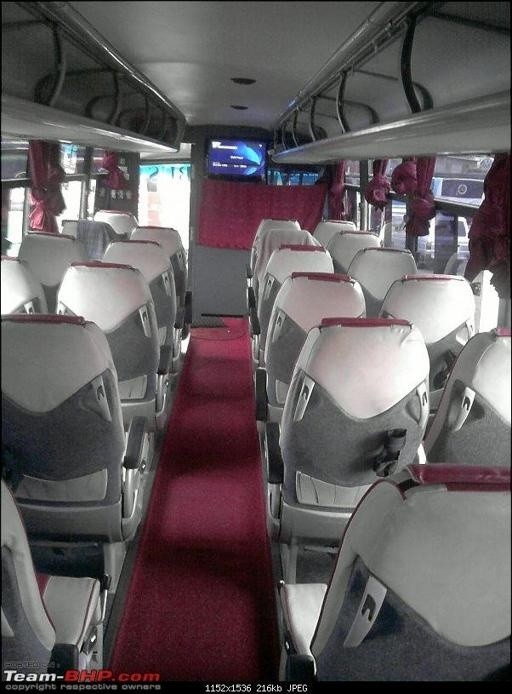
[271,2,511,164]
[1,2,188,153]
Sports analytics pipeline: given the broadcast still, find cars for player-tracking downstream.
[358,176,488,264]
[287,172,319,187]
[9,169,26,212]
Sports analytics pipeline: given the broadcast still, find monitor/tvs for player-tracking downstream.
[206,136,268,178]
